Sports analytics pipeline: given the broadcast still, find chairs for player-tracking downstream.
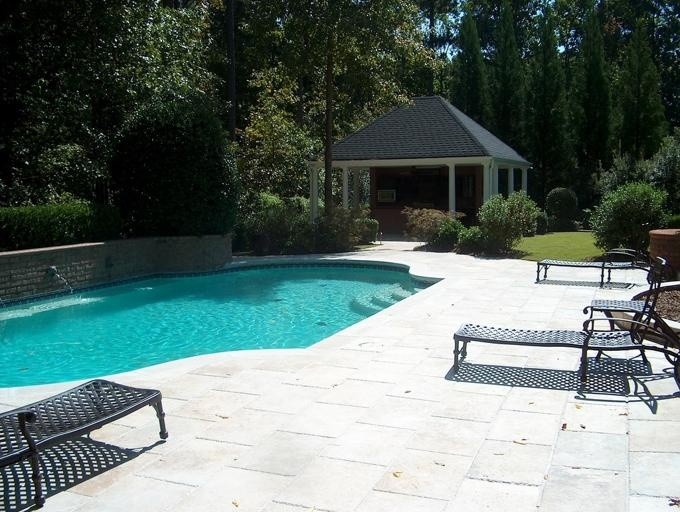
[0,378,168,508]
[453,248,680,383]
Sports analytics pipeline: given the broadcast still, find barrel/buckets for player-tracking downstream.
[649,228,680,280]
